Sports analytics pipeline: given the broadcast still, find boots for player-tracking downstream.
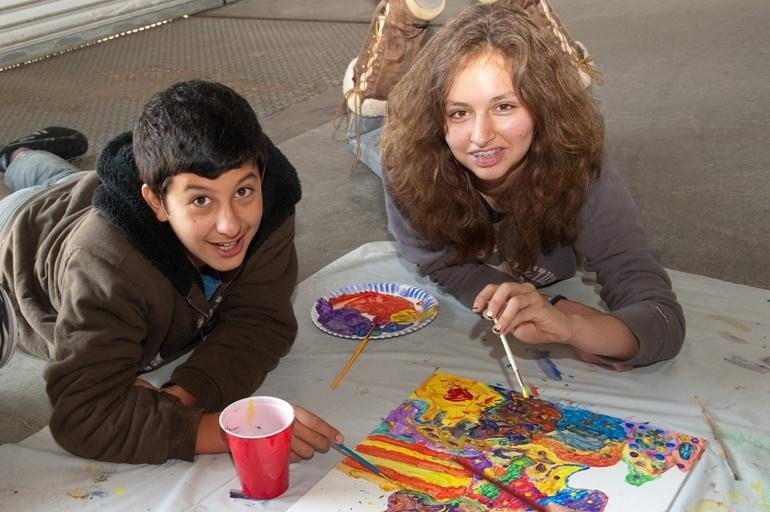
[528,1,594,89]
[343,0,445,118]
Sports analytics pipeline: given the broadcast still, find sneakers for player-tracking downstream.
[0,127,88,172]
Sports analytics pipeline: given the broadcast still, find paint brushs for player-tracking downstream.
[452,454,547,512]
[332,322,377,388]
[336,442,396,485]
[487,301,531,399]
[692,395,739,481]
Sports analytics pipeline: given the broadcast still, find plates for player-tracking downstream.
[312,282,441,340]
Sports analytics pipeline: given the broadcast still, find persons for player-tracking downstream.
[0,77,347,468]
[340,1,690,373]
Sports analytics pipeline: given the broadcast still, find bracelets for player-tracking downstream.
[545,294,568,307]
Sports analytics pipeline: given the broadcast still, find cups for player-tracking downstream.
[218,394,296,502]
